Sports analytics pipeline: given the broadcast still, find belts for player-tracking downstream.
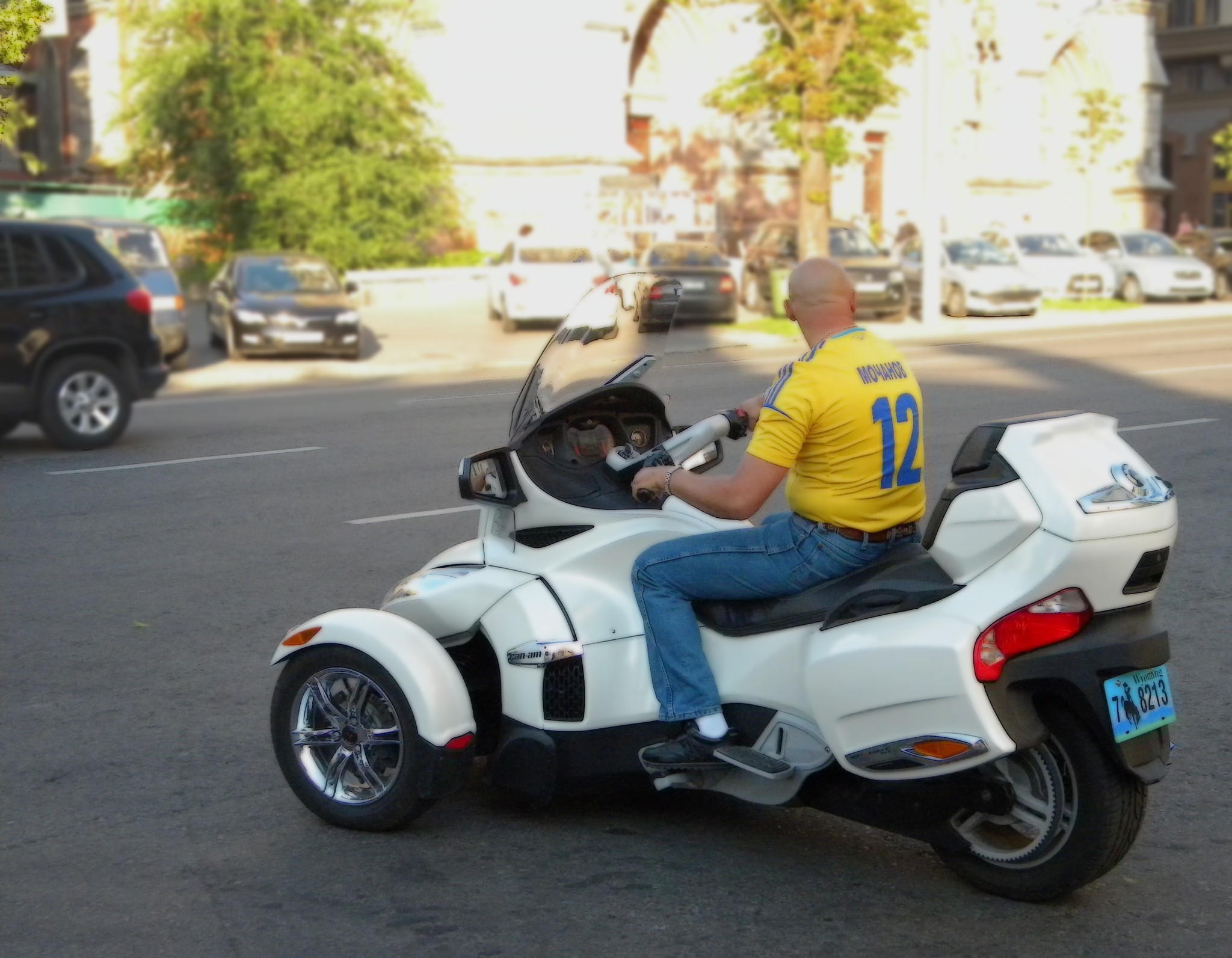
[804,518,916,543]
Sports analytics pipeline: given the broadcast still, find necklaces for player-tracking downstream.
[817,324,857,343]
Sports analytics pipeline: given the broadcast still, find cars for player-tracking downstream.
[206,251,363,362]
[1075,228,1217,303]
[481,237,617,334]
[1014,232,1117,303]
[741,216,907,319]
[633,240,741,330]
[885,234,1042,317]
[1170,226,1232,298]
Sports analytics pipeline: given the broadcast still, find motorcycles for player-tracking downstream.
[267,267,1180,903]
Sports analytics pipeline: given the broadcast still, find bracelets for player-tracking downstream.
[664,465,684,496]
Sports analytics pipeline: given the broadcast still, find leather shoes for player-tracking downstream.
[642,722,738,769]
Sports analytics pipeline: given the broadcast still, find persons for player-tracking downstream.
[1177,212,1206,232]
[630,259,927,771]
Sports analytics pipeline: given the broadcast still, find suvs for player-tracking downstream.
[1,221,170,450]
[94,226,188,362]
